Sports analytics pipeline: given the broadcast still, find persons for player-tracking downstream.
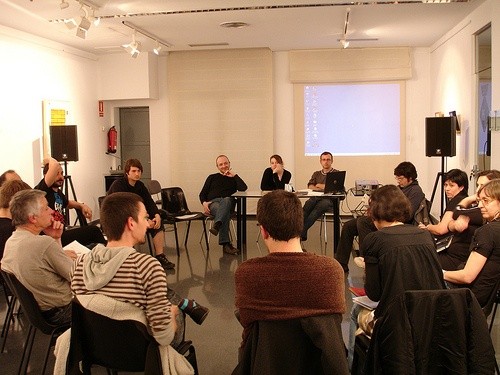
[107,159,176,270]
[0,156,107,259]
[1,190,77,327]
[260,155,292,191]
[351,184,448,336]
[347,169,500,316]
[198,155,248,255]
[231,190,351,375]
[301,152,341,242]
[72,192,209,375]
[334,161,425,273]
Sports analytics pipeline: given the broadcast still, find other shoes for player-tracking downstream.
[188,299,208,325]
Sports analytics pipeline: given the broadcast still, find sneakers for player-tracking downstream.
[209,222,220,236]
[155,254,175,268]
[223,243,240,254]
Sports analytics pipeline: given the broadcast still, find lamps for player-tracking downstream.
[152,41,162,56]
[77,3,92,39]
[339,39,349,48]
[59,0,69,10]
[123,29,142,59]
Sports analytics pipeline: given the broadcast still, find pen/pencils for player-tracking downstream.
[229,168,232,171]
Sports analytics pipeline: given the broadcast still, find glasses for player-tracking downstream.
[476,197,494,206]
[394,176,404,181]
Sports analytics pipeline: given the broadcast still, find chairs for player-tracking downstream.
[0,270,72,375]
[66,294,199,375]
[322,199,344,244]
[231,313,351,375]
[351,275,500,375]
[97,180,238,257]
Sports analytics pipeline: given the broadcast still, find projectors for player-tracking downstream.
[354,180,379,195]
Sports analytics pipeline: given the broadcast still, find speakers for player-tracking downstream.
[425,117,457,157]
[49,125,79,162]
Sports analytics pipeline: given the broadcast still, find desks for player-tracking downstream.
[231,188,346,257]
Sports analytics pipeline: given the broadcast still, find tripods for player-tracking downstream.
[430,156,446,219]
[60,161,84,227]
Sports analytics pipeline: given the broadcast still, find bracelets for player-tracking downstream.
[426,225,429,229]
[442,269,447,274]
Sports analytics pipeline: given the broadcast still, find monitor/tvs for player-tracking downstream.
[324,170,346,195]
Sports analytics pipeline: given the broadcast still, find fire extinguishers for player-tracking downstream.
[108,125,118,153]
